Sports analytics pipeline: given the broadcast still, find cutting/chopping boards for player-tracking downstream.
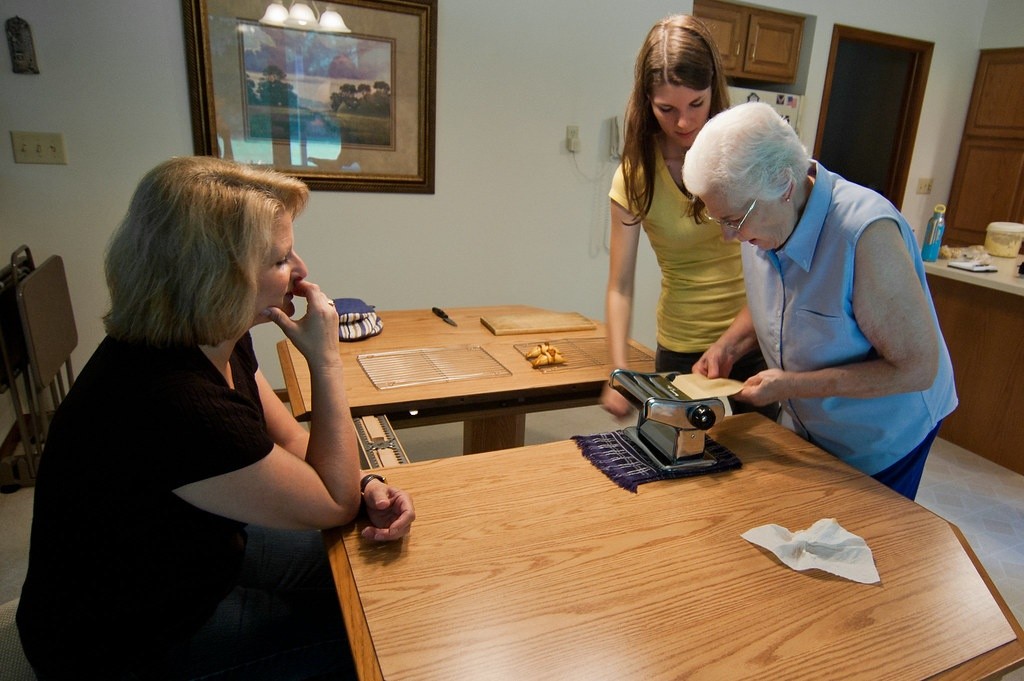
[480,311,597,336]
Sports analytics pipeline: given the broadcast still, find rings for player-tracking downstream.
[328,299,334,306]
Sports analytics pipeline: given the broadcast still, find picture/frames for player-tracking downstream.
[182,0,438,196]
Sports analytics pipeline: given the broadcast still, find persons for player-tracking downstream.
[602,13,781,426]
[681,101,960,501]
[14,157,415,681]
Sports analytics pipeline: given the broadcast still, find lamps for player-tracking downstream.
[259,0,352,35]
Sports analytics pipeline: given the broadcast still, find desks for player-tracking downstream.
[276,307,656,457]
[323,411,1023,681]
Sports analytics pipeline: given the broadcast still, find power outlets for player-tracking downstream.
[566,125,580,148]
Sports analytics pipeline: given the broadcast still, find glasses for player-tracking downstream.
[702,182,775,234]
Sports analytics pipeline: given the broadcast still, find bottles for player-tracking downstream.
[921,205,946,262]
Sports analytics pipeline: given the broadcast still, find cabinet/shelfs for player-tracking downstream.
[941,45,1024,247]
[693,0,805,85]
[920,245,1024,477]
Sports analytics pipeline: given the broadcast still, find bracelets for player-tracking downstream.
[360,474,388,503]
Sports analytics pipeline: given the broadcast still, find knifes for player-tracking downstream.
[431,307,458,326]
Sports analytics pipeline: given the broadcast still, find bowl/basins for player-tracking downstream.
[985,221,1024,259]
